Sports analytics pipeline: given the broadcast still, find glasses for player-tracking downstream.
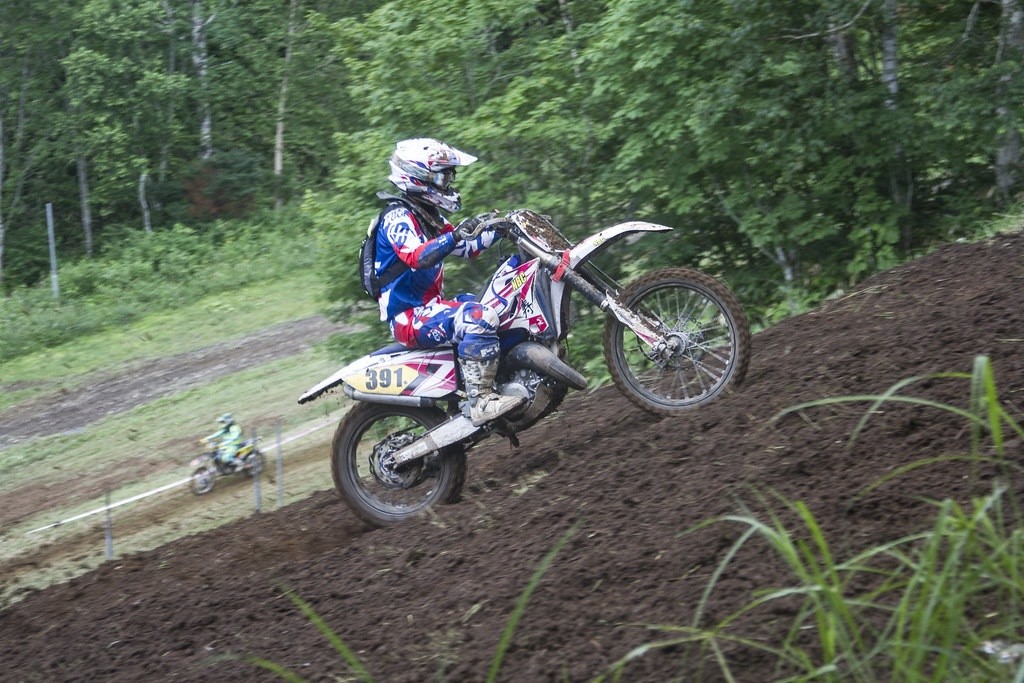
[434,171,457,190]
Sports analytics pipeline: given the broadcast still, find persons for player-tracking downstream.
[359,137,527,426]
[201,412,246,473]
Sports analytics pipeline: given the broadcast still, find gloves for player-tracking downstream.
[453,217,484,242]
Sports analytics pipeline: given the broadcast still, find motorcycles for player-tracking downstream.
[189,436,268,497]
[298,207,754,530]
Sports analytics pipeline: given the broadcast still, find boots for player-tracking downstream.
[233,458,246,472]
[458,349,528,428]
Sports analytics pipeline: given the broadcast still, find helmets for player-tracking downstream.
[216,413,233,426]
[388,137,477,214]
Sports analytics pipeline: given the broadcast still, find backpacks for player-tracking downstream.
[359,205,433,302]
